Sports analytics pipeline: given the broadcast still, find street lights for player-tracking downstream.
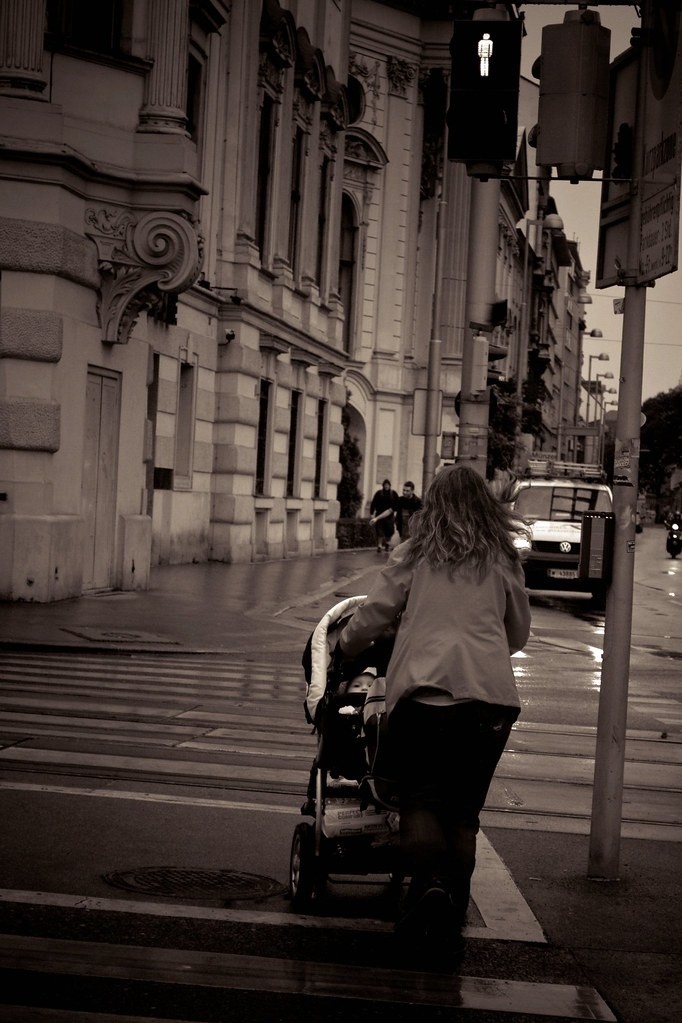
[572,328,604,461]
[514,214,566,475]
[593,371,614,465]
[597,388,616,466]
[601,401,619,468]
[583,354,610,461]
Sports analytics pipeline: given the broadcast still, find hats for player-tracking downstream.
[382,479,390,486]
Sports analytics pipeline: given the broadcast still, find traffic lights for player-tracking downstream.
[447,17,521,168]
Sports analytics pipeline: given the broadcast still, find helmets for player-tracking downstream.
[674,510,681,519]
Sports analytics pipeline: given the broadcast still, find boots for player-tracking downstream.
[408,844,475,955]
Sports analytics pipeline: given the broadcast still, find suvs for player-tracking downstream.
[494,460,617,607]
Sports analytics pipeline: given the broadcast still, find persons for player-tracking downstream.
[331,467,532,937]
[370,478,399,551]
[339,667,377,763]
[666,510,682,530]
[370,481,421,537]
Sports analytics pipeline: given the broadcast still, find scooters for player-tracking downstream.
[663,519,682,560]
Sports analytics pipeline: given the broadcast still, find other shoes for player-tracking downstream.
[385,544,389,550]
[378,547,382,552]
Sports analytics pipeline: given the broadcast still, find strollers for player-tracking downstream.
[290,594,420,900]
